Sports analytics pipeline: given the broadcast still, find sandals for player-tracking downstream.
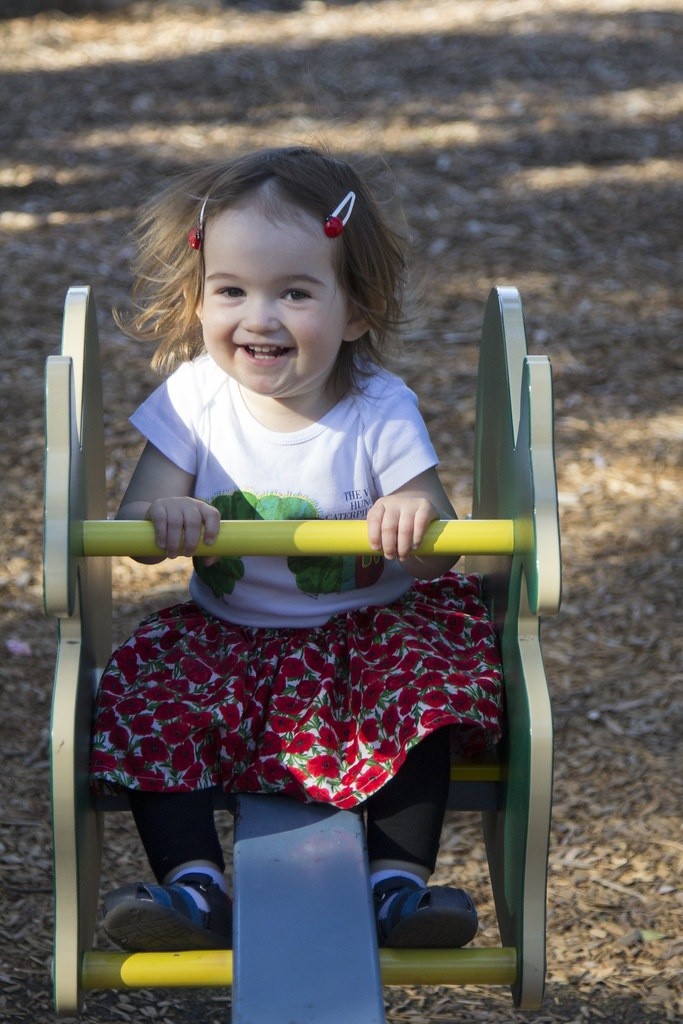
[100,873,234,949]
[371,875,478,948]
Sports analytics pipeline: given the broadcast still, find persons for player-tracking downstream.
[89,146,506,952]
